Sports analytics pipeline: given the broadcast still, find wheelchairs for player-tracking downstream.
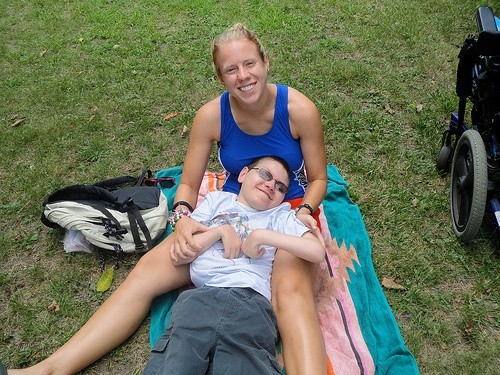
[435,6,500,244]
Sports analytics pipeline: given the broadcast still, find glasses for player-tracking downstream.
[144,177,176,189]
[250,168,288,194]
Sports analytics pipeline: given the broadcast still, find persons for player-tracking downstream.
[1,22,328,375]
[142,155,325,375]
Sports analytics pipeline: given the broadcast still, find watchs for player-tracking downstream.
[294,204,313,215]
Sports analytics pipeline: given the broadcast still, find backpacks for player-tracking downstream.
[41,169,168,254]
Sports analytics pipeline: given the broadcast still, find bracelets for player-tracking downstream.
[170,209,192,232]
[171,200,194,213]
